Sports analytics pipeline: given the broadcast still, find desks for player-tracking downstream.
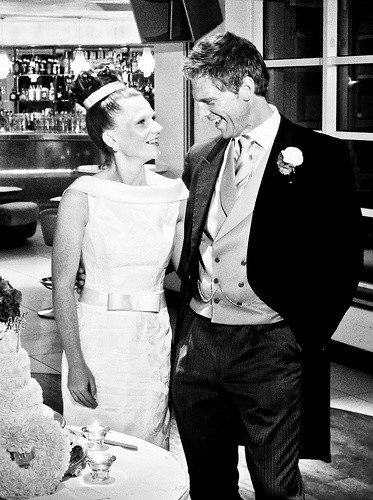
[30,423,190,500]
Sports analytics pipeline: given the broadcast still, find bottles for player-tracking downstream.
[4,108,86,133]
[12,55,60,75]
[18,79,74,101]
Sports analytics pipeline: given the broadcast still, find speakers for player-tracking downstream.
[130,0,223,44]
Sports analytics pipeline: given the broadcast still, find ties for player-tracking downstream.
[234,136,258,193]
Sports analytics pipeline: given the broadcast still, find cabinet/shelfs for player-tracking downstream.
[15,48,153,113]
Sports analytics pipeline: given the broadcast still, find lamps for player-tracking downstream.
[137,45,156,77]
[69,19,91,72]
[0,17,14,78]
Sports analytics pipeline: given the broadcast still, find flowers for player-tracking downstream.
[277,145,303,175]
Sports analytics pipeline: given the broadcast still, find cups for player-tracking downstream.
[86,454,116,481]
[81,425,110,449]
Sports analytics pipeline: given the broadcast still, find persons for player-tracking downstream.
[76,31,364,500]
[51,71,190,451]
